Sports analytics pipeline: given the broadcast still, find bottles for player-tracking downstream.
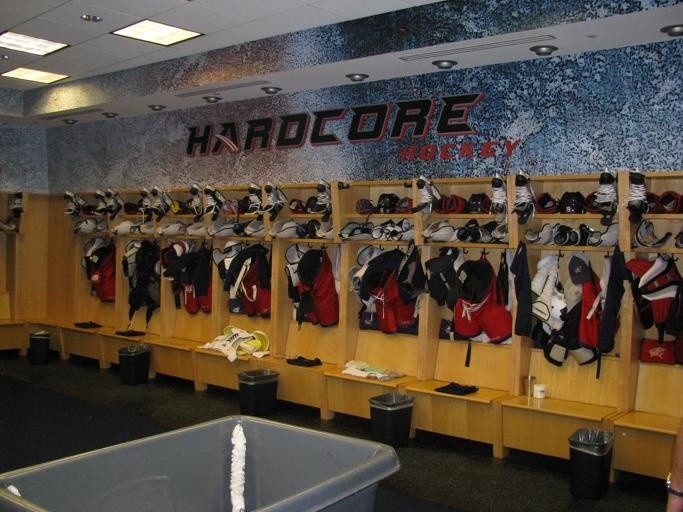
[533,384,545,399]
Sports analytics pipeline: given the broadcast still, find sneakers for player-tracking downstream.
[248,185,284,222]
[10,199,23,211]
[627,174,649,224]
[190,187,222,221]
[513,174,534,225]
[311,184,329,214]
[62,193,80,217]
[94,191,122,221]
[491,178,506,213]
[138,189,169,222]
[417,179,438,215]
[595,173,617,227]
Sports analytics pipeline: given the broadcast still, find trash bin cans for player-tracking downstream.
[568,428,616,499]
[238,369,279,417]
[29,329,50,364]
[118,345,150,385]
[368,392,413,446]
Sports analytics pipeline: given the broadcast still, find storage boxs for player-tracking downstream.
[0,413,401,512]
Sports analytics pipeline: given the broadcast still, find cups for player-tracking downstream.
[524,376,536,396]
